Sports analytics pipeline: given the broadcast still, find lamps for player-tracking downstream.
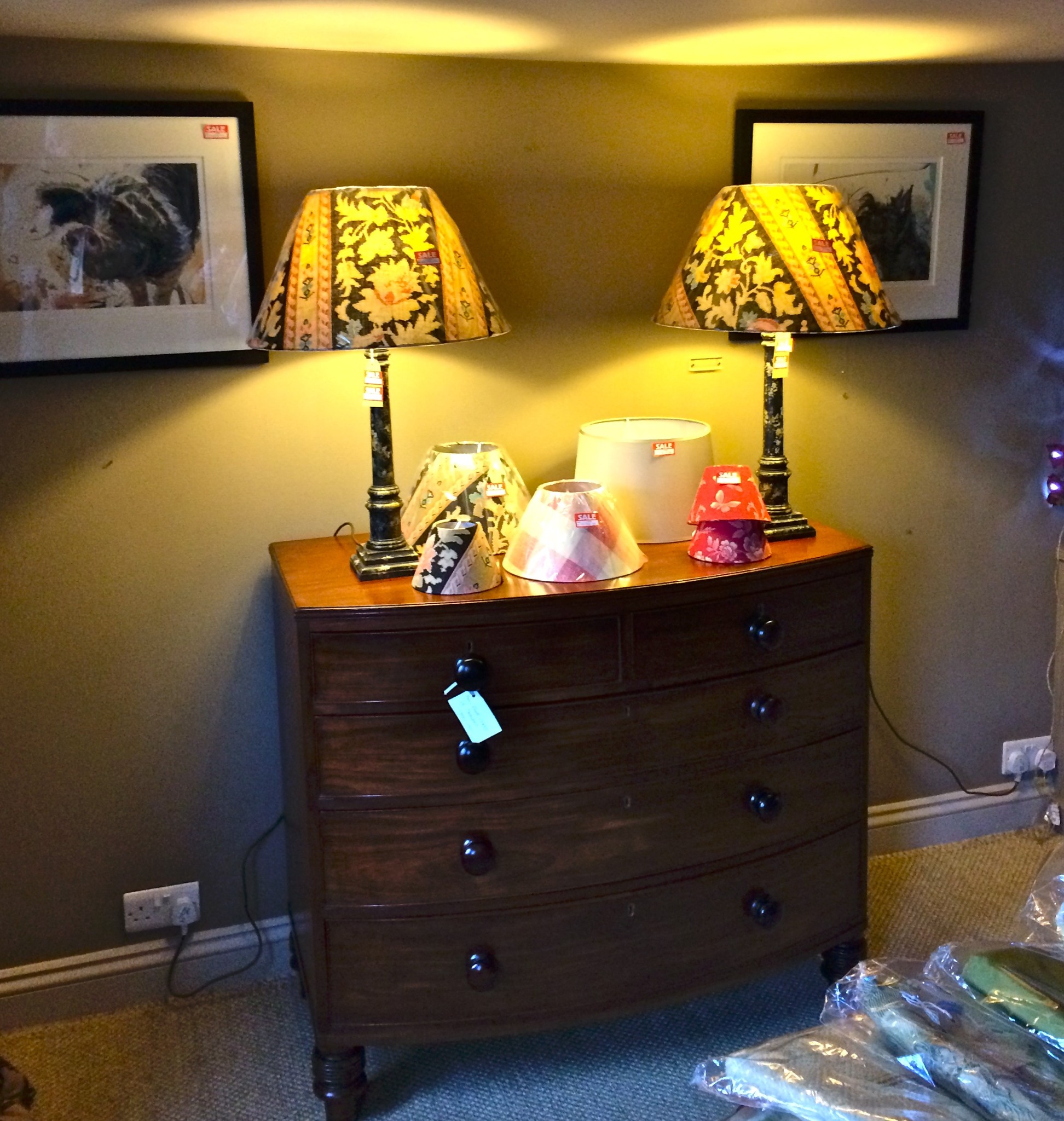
[650,183,903,541]
[249,191,511,581]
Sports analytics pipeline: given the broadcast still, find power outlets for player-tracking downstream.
[123,881,200,932]
[1001,736,1054,777]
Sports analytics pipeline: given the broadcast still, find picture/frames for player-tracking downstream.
[0,100,269,376]
[727,106,985,338]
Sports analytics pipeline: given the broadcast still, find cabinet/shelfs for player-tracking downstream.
[259,508,873,1121]
